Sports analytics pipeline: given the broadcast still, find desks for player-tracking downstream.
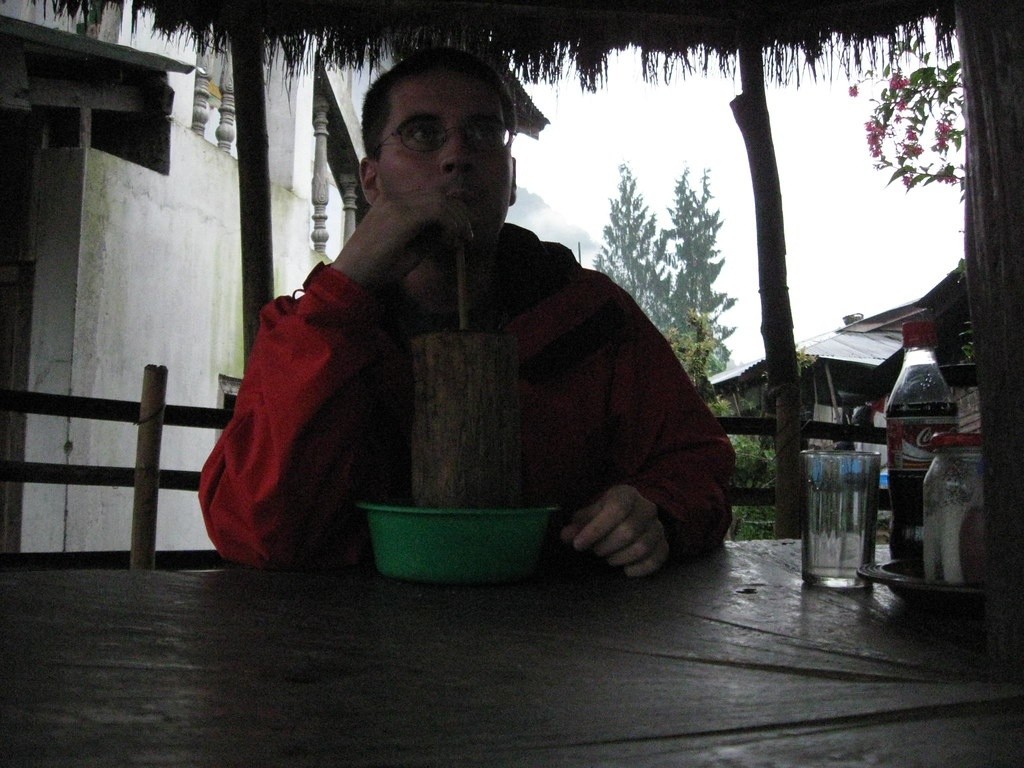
[1,542,1024,768]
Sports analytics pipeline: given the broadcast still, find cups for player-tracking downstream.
[797,448,880,590]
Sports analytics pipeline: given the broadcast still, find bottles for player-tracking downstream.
[887,322,958,562]
[921,429,993,589]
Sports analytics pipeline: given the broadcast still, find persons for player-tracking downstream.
[198,46,736,581]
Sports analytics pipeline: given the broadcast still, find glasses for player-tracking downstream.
[372,113,515,159]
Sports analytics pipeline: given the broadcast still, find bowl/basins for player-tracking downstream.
[353,495,560,582]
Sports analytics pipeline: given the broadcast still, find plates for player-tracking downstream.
[857,558,992,618]
